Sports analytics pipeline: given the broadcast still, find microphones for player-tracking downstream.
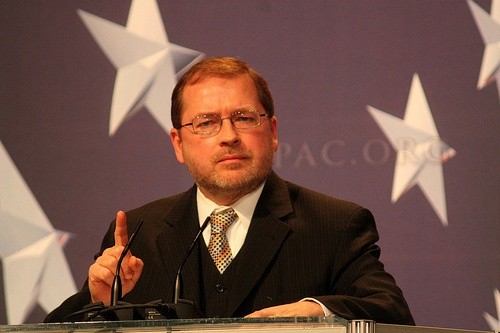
[84,216,211,322]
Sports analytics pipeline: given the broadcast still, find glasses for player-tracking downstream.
[179,108,268,135]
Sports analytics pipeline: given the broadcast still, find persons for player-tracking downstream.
[43,56,417,326]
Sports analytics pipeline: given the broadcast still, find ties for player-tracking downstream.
[207,207,237,275]
